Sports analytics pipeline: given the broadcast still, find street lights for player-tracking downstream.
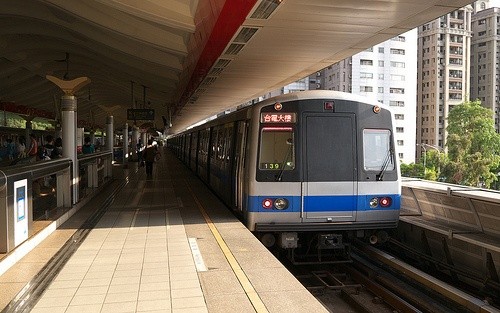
[416,144,426,167]
[423,144,440,173]
[434,145,446,157]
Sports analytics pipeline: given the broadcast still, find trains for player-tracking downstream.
[0,126,106,157]
[166,90,402,274]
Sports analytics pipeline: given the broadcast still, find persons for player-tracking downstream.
[37,135,56,157]
[26,134,37,158]
[0,135,27,158]
[96,137,101,145]
[82,136,95,154]
[142,139,156,177]
[51,137,62,160]
[158,138,168,150]
[150,136,158,167]
[136,138,144,167]
[113,139,123,146]
